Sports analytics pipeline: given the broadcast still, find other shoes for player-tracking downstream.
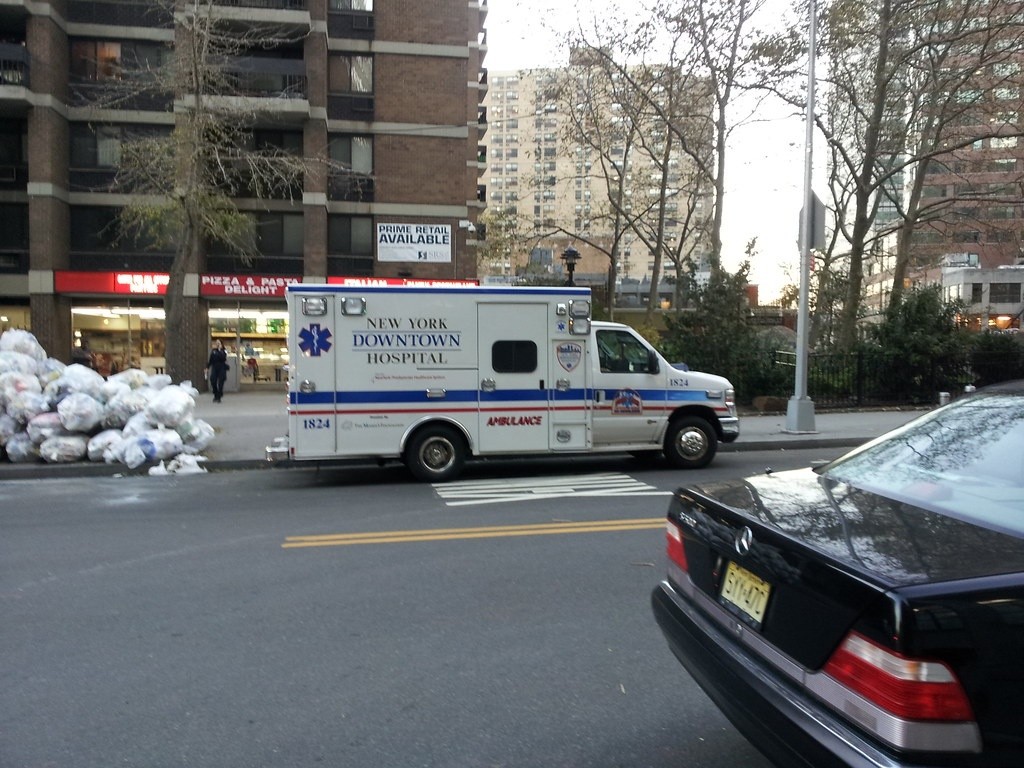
[213,396,220,402]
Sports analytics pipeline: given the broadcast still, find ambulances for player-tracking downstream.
[266,281,739,483]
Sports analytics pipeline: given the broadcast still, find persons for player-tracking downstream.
[69,336,118,381]
[244,342,255,356]
[204,338,226,403]
[248,357,256,382]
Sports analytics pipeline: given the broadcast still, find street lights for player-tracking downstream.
[560,242,582,286]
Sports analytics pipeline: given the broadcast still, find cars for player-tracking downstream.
[651,380,1024,768]
